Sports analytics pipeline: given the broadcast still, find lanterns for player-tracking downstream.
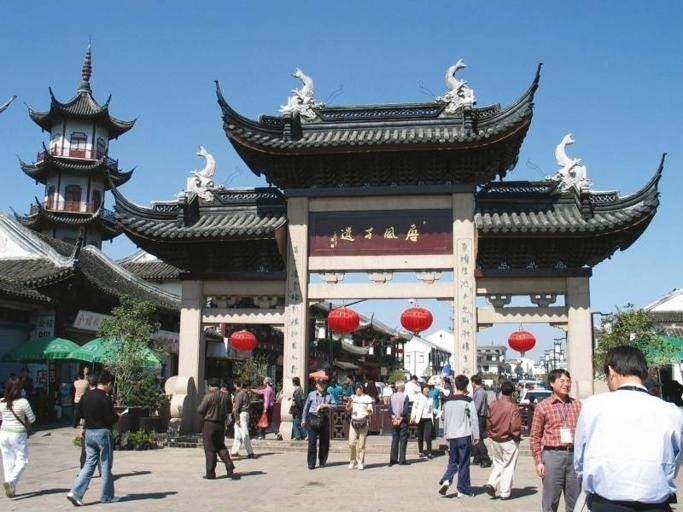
[400,304,433,336]
[507,328,536,357]
[230,330,257,356]
[327,305,360,338]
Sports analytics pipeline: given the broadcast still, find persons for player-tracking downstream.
[291,370,373,471]
[437,374,481,498]
[72,371,102,478]
[374,376,537,468]
[65,372,123,507]
[196,375,283,482]
[529,368,582,511]
[573,345,683,512]
[0,363,104,429]
[481,379,522,501]
[0,373,37,498]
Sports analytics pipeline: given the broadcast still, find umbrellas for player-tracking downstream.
[629,331,683,400]
[66,331,163,403]
[0,335,98,395]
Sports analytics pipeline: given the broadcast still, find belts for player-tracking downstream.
[543,446,574,452]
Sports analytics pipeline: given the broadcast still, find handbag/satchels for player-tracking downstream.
[305,415,324,430]
[352,418,366,428]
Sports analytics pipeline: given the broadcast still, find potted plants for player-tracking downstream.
[231,350,272,438]
[73,293,173,451]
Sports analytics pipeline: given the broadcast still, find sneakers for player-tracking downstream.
[231,452,253,458]
[4,481,15,498]
[486,485,510,500]
[348,464,364,470]
[67,492,121,506]
[439,480,474,497]
